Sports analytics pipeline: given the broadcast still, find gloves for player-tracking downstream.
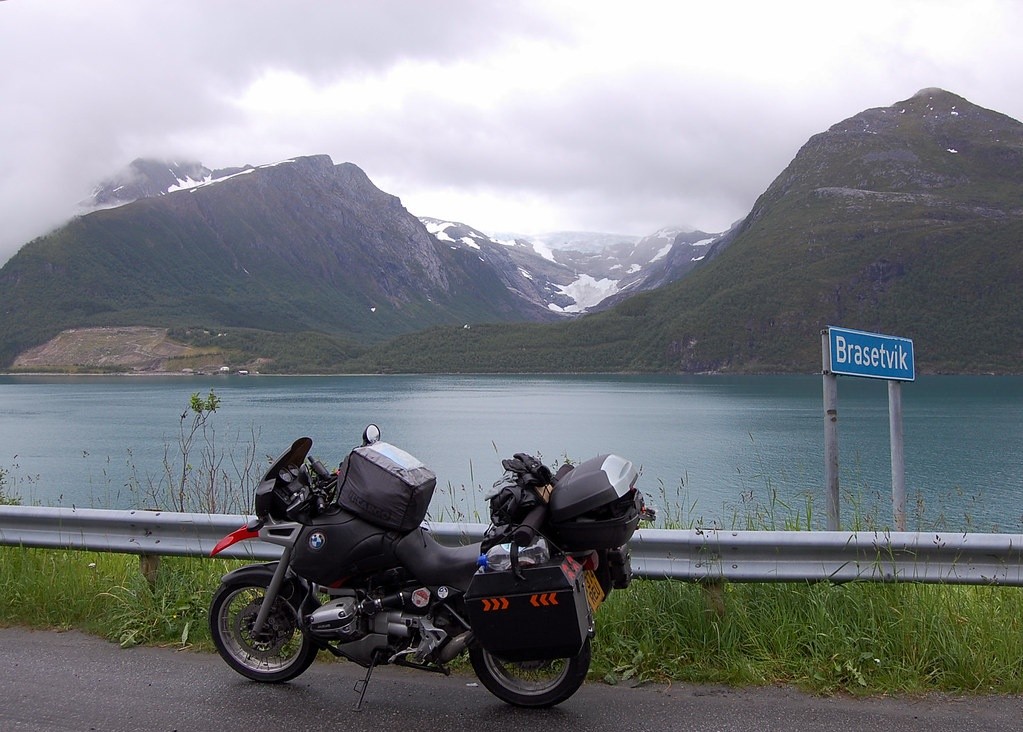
[513,453,551,483]
[501,458,543,488]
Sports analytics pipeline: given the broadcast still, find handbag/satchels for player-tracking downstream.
[485,468,536,527]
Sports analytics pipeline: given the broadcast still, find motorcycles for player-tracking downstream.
[208,423,658,711]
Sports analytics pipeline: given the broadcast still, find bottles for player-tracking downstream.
[477,536,549,570]
